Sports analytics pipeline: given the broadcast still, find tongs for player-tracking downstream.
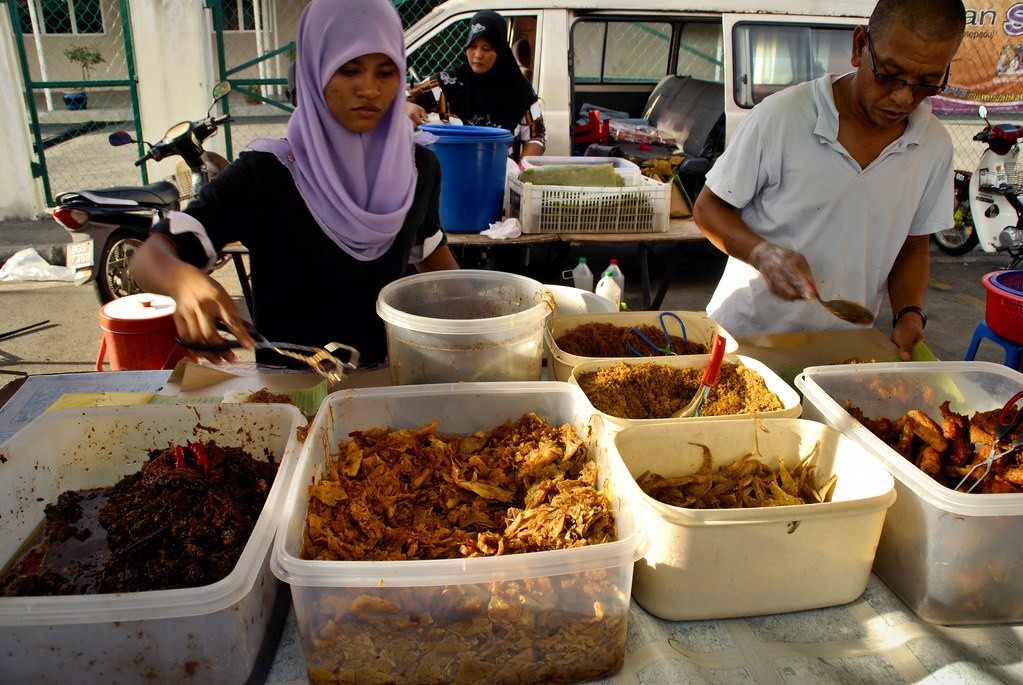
[176,315,359,383]
[953,391,1023,495]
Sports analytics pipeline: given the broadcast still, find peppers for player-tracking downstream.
[174,438,211,473]
[205,493,214,514]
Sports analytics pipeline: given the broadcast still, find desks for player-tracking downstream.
[440,214,706,315]
[1,328,1023,685]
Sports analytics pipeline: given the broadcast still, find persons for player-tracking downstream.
[692,0,966,362]
[405,10,546,168]
[129,0,498,371]
[892,306,927,330]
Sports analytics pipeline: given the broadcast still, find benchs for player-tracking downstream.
[581,72,725,172]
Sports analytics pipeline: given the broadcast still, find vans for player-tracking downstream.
[404,0,874,160]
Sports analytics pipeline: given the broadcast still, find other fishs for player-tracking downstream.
[638,440,838,509]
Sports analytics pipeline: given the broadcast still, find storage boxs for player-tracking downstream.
[0,400,303,684]
[519,155,641,172]
[270,380,649,685]
[791,361,1023,626]
[506,170,673,234]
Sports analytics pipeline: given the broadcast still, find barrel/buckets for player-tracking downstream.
[539,281,617,362]
[96,292,196,373]
[375,269,553,388]
[419,122,514,236]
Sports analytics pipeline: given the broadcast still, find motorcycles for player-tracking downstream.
[51,80,231,306]
[930,105,1023,270]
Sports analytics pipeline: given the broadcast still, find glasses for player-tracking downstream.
[867,28,950,97]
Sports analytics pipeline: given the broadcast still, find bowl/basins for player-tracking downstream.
[981,270,1023,344]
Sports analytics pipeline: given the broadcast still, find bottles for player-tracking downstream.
[574,256,594,292]
[596,272,622,314]
[602,259,623,301]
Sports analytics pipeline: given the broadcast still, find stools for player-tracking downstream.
[964,318,1023,371]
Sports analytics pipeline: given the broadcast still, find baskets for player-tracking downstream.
[507,171,671,234]
